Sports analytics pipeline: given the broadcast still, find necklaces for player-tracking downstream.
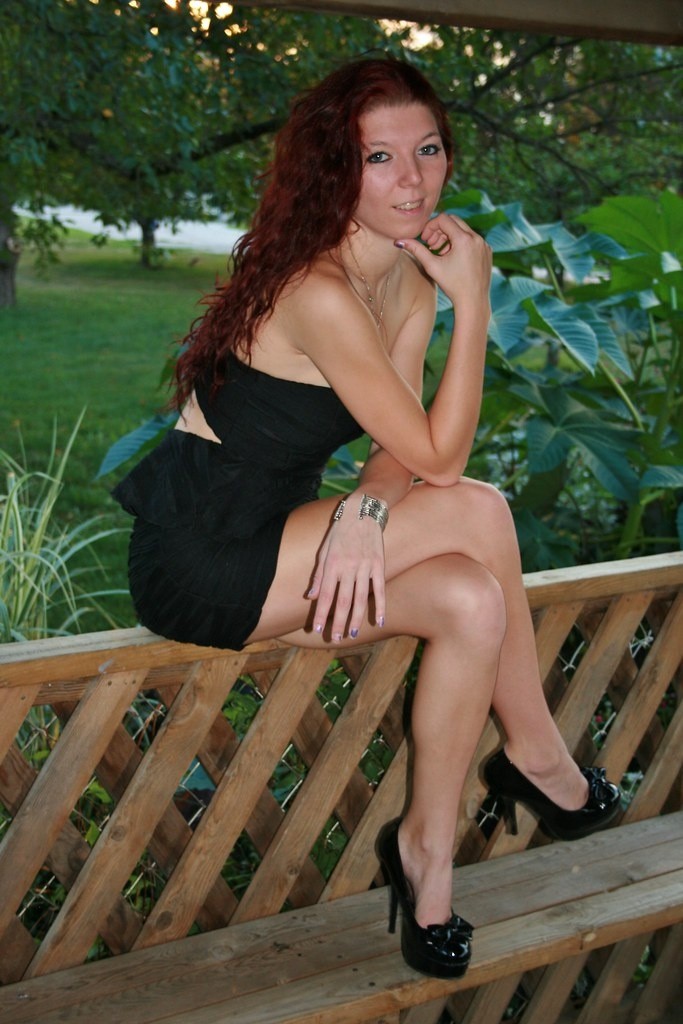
[351,251,389,329]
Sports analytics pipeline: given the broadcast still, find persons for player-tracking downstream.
[109,59,622,978]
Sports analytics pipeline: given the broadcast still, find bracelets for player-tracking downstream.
[334,495,388,534]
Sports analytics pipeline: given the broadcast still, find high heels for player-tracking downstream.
[375,816,474,978]
[482,746,623,840]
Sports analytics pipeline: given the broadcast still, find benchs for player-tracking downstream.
[0,810,683,1024]
